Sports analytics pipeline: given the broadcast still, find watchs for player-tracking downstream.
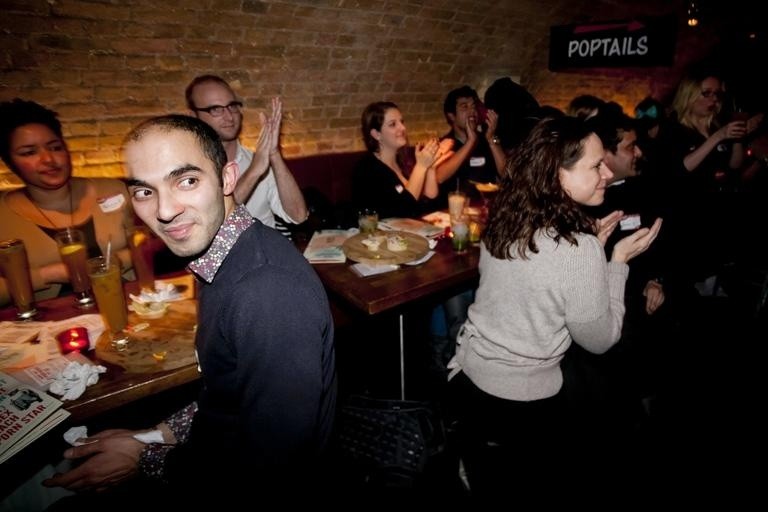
[140,442,165,480]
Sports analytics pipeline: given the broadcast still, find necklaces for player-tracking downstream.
[28,182,73,229]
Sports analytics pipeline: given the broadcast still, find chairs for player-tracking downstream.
[331,397,427,512]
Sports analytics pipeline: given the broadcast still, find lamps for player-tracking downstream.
[684,0,699,27]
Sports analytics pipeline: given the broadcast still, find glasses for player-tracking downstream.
[195,102,243,117]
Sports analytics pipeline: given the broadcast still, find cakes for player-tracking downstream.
[386,235,408,251]
[128,280,181,318]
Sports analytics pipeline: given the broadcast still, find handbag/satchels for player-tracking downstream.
[335,396,446,476]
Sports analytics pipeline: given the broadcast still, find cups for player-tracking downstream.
[0,238,37,320]
[356,207,380,234]
[53,228,93,308]
[734,104,749,143]
[125,224,153,296]
[451,213,470,255]
[447,191,465,216]
[469,219,481,243]
[84,256,134,348]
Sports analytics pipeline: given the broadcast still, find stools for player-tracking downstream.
[445,373,574,512]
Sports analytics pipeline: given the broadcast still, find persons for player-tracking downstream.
[186,75,309,239]
[0,98,137,301]
[39,114,335,512]
[352,73,767,502]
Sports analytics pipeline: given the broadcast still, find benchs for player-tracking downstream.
[121,144,429,273]
